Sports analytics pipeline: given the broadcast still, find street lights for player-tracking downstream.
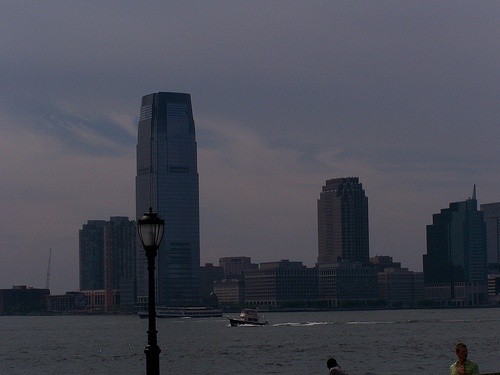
[137,206,166,375]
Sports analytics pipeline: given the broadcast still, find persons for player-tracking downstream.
[325,358,342,375]
[448,343,480,375]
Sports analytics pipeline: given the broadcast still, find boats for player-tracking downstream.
[229,308,268,327]
